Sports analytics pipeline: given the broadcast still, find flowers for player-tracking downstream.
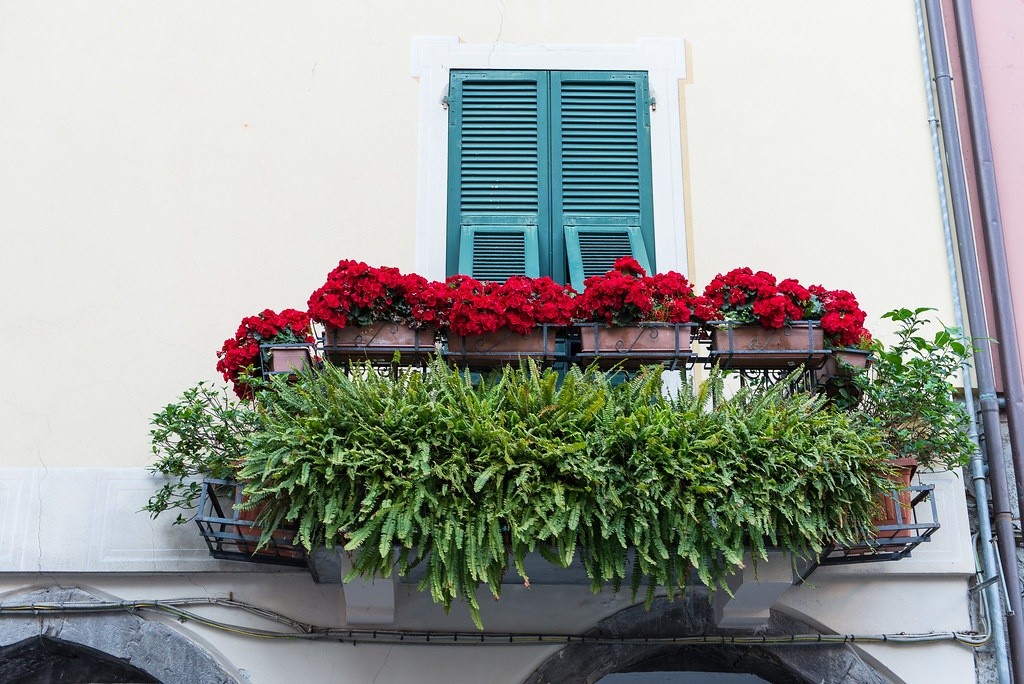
[217,256,878,398]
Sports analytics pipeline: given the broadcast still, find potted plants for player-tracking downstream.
[135,307,1015,628]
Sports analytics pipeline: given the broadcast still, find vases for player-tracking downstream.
[709,328,824,368]
[322,321,437,364]
[578,326,692,370]
[813,349,869,391]
[447,324,556,373]
[266,346,312,377]
